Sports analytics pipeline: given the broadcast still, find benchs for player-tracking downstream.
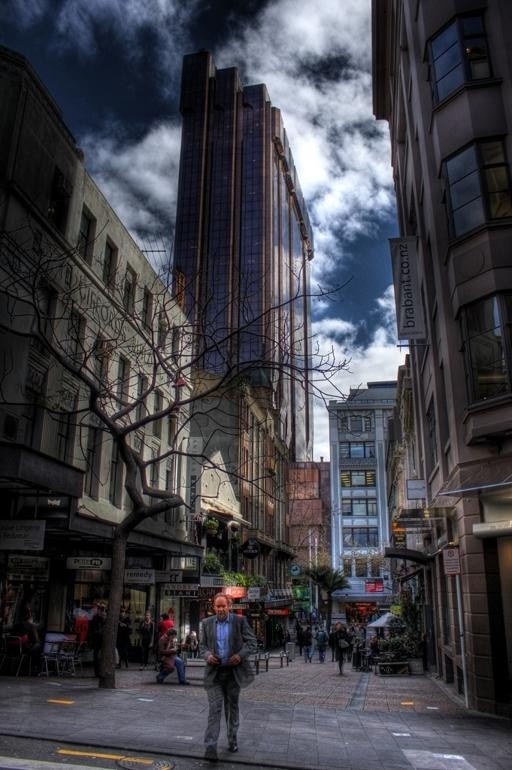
[182,650,292,674]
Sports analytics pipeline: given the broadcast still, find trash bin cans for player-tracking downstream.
[286,642,296,661]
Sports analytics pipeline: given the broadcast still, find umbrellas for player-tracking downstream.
[366,611,407,631]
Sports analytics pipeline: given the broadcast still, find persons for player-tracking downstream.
[4,600,106,677]
[276,618,362,674]
[199,593,257,760]
[116,609,198,684]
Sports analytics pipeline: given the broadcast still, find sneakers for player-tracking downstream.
[229,741,238,752]
[157,675,162,683]
[179,681,188,684]
[205,747,218,761]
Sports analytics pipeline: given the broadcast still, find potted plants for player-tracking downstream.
[204,519,220,534]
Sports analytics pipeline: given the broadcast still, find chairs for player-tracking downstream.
[0,632,90,678]
